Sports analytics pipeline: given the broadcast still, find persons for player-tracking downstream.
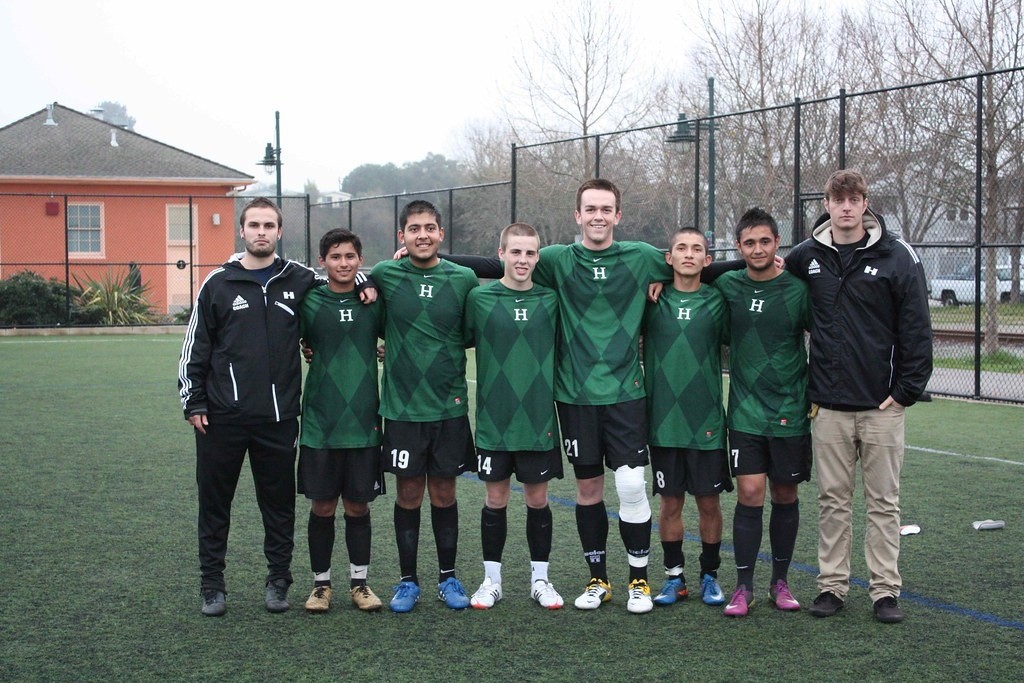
[297,229,384,611]
[301,201,480,612]
[178,195,377,615]
[641,227,735,605]
[782,170,934,624]
[649,208,810,617]
[379,224,562,610]
[392,179,784,613]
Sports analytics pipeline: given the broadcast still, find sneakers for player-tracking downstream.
[264,579,289,612]
[470,576,504,609]
[874,597,904,623]
[201,588,226,615]
[305,585,333,611]
[349,584,383,611]
[653,576,688,605]
[574,577,613,610]
[699,574,726,606]
[807,591,844,617]
[389,582,421,613]
[530,581,564,610]
[437,576,470,609]
[768,579,800,612]
[723,584,756,616]
[626,579,653,614]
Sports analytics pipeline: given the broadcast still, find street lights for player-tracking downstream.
[255,110,282,257]
[665,77,716,264]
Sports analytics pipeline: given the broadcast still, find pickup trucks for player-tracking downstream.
[930,265,1024,307]
